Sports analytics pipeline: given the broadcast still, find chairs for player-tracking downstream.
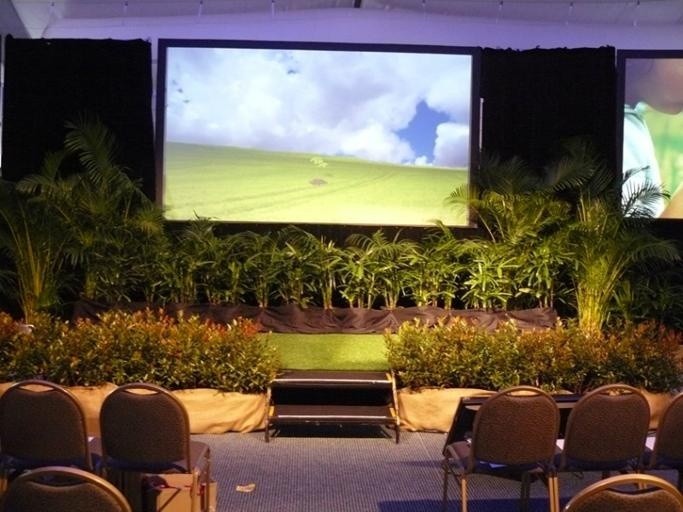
[0,378,211,512]
[441,383,683,512]
